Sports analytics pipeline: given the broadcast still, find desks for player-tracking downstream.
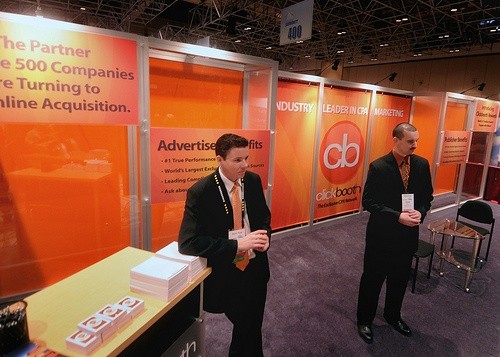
[0,245,213,357]
[427,219,485,293]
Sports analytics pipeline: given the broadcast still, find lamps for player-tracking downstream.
[234,12,500,94]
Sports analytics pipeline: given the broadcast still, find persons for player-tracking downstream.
[356,123,434,343]
[178,134,272,357]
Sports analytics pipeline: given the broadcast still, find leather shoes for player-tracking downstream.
[386,318,412,337]
[357,322,374,343]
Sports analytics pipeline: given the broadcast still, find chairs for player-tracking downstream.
[452,200,495,273]
[409,240,435,294]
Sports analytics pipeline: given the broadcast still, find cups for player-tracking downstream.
[0,299,29,357]
[443,217,457,233]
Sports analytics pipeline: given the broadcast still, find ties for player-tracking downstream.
[401,157,410,191]
[233,182,249,271]
